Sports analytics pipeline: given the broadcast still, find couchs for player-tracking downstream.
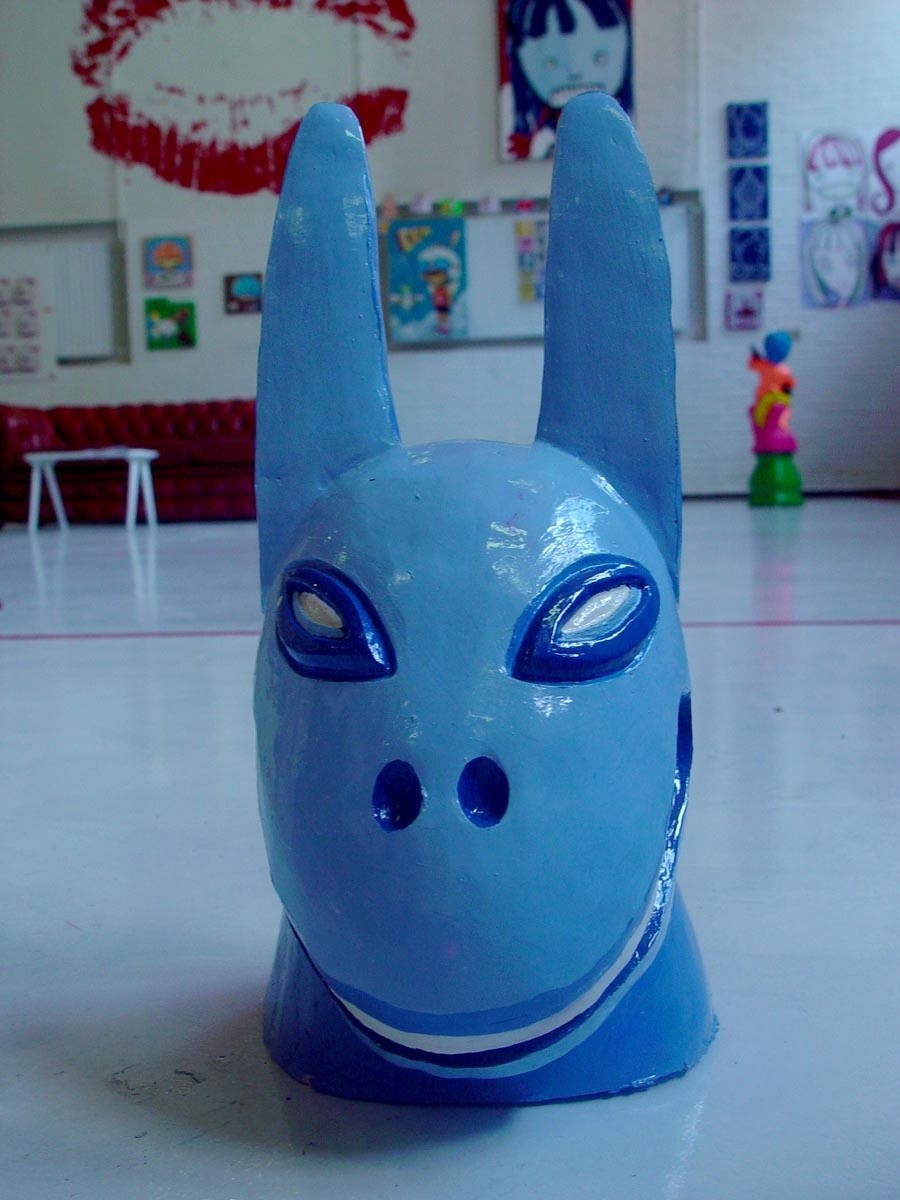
[0,396,255,532]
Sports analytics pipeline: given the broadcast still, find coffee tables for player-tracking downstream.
[19,446,162,535]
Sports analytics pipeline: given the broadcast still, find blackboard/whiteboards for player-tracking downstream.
[464,189,706,343]
[0,221,128,362]
